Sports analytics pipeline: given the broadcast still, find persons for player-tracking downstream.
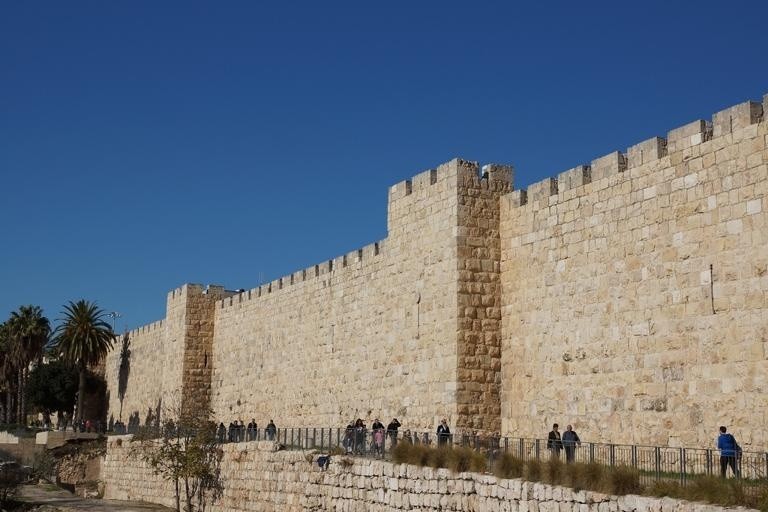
[717,426,742,479]
[436,418,450,446]
[265,419,277,441]
[72,418,91,433]
[217,418,258,443]
[342,418,401,455]
[547,424,581,464]
[30,419,40,427]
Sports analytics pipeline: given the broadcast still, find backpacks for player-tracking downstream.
[730,435,742,459]
